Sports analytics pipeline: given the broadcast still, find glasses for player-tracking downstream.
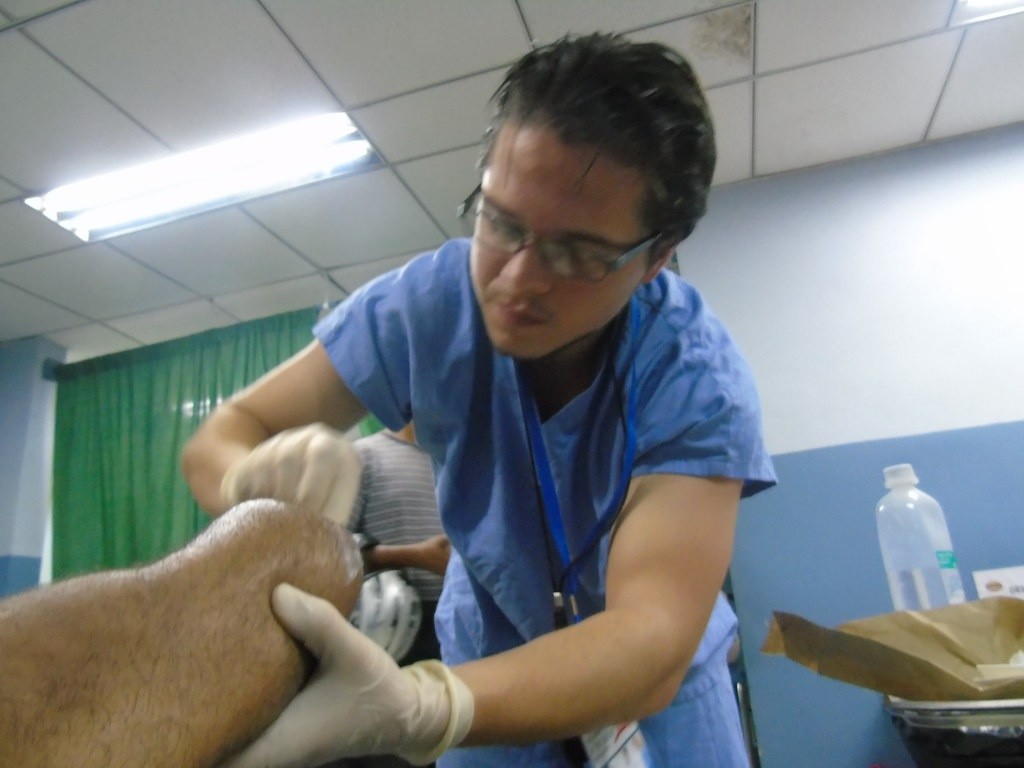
[455,183,664,282]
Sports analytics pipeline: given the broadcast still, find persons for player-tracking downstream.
[183,35,778,768]
[0,497,364,768]
[347,422,451,666]
